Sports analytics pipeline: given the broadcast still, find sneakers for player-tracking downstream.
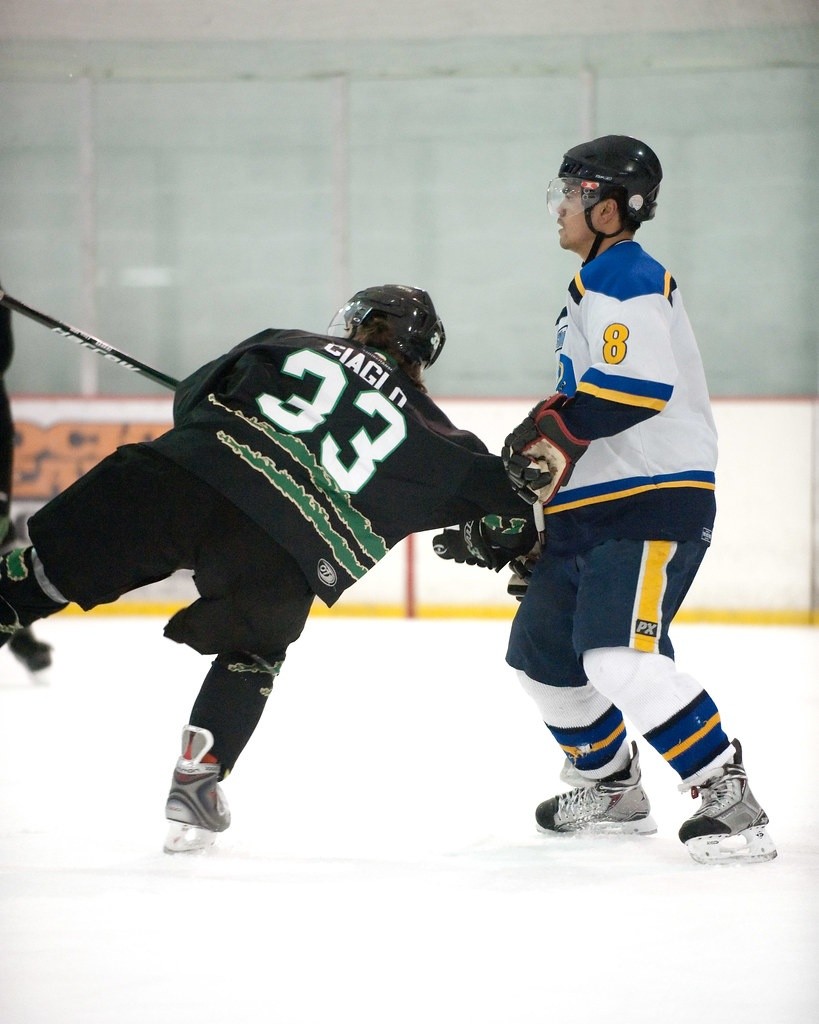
[534,739,657,836]
[678,738,777,863]
[163,726,229,852]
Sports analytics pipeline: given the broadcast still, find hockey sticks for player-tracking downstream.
[511,453,547,551]
[2,291,182,392]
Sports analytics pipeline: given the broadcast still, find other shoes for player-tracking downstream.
[11,632,54,678]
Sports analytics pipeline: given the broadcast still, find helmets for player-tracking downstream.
[557,135,663,222]
[344,284,446,369]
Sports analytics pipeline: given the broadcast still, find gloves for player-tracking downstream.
[453,537,520,573]
[502,394,592,508]
[433,519,495,567]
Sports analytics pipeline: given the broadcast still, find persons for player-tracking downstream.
[500,135,769,843]
[0,285,53,673]
[0,284,549,832]
